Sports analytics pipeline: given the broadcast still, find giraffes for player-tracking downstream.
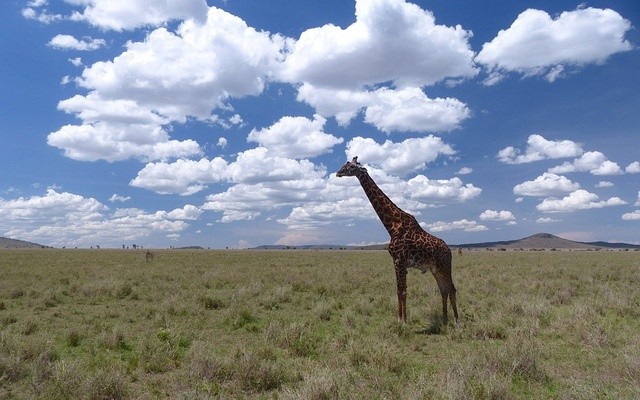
[336,155,459,325]
